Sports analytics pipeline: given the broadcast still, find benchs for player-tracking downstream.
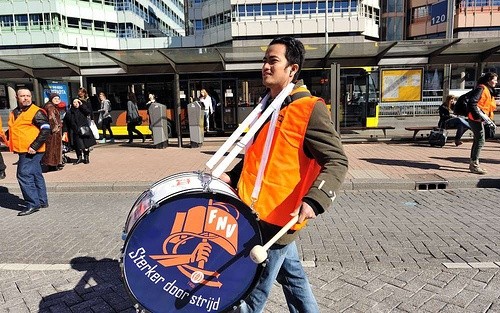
[339,126,395,139]
[404,126,438,141]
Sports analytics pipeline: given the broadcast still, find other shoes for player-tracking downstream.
[470,163,485,173]
[455,140,463,146]
[0,170,5,179]
[128,140,133,144]
[142,136,145,143]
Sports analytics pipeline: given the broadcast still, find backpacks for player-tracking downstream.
[455,87,483,116]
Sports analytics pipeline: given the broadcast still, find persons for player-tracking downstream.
[42,93,62,171]
[144,91,159,141]
[77,88,93,122]
[468,72,498,174]
[55,101,66,123]
[0,87,51,216]
[98,92,115,144]
[438,95,470,147]
[193,88,219,133]
[62,98,97,164]
[126,92,146,143]
[218,36,348,313]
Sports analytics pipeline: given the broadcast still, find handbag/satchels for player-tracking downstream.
[62,132,68,142]
[430,128,447,147]
[88,120,100,140]
[80,126,90,136]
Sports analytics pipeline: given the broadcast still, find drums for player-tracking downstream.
[118,171,266,313]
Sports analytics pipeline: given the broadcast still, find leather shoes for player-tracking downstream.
[39,204,48,208]
[19,205,39,216]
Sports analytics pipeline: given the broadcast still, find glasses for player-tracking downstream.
[274,36,298,54]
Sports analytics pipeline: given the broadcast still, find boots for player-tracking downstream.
[84,154,88,164]
[109,138,114,143]
[99,138,106,144]
[76,153,82,163]
